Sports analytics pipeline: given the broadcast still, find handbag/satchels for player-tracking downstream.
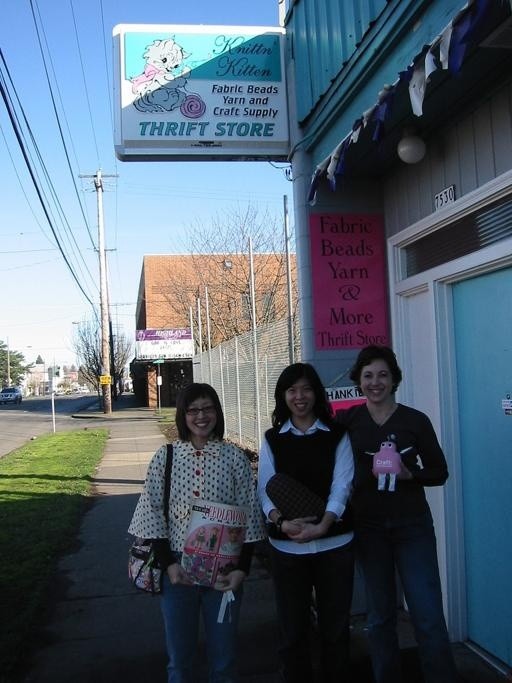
[126,533,167,595]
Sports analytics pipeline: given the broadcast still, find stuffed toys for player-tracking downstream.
[370,435,409,495]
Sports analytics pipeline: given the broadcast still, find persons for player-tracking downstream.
[220,526,243,554]
[332,346,457,682]
[260,363,354,681]
[207,525,219,551]
[127,382,268,682]
[195,525,206,549]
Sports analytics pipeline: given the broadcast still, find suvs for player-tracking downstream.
[0,386,22,403]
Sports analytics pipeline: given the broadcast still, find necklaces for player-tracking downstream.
[365,400,395,429]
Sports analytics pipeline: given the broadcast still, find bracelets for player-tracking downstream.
[275,515,287,531]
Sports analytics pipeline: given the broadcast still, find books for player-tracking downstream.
[180,498,250,593]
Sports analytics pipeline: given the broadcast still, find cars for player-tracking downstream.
[49,386,91,396]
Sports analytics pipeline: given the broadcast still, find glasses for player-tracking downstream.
[184,403,218,415]
